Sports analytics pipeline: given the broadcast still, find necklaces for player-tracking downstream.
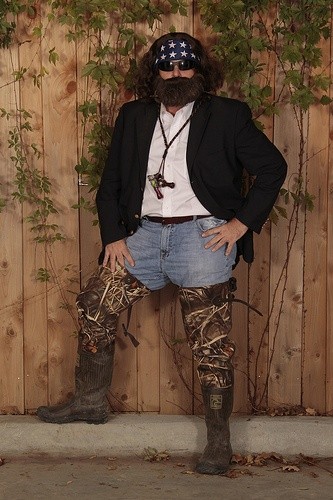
[147,112,192,200]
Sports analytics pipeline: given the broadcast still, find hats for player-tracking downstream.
[150,32,202,67]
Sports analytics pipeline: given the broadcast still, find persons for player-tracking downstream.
[33,32,289,477]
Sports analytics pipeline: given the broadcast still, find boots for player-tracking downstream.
[194,384,234,474]
[35,345,115,423]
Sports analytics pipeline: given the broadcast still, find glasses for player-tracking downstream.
[156,59,197,71]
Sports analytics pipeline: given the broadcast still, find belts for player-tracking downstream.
[144,215,214,225]
